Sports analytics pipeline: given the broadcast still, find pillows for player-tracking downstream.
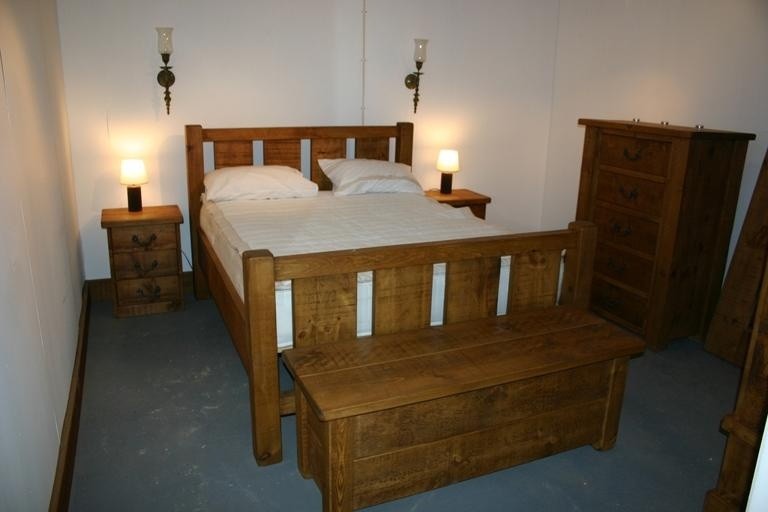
[317,158,423,195]
[204,164,319,202]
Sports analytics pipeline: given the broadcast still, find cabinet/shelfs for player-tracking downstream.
[576,117,757,347]
[704,270,767,512]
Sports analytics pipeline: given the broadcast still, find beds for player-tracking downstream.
[182,121,599,465]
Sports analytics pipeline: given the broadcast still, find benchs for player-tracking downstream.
[283,305,648,512]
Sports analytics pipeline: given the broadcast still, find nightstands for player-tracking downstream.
[425,189,490,219]
[101,203,185,318]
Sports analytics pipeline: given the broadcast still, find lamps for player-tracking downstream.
[438,149,460,192]
[122,159,146,211]
[402,37,430,113]
[152,26,179,116]
